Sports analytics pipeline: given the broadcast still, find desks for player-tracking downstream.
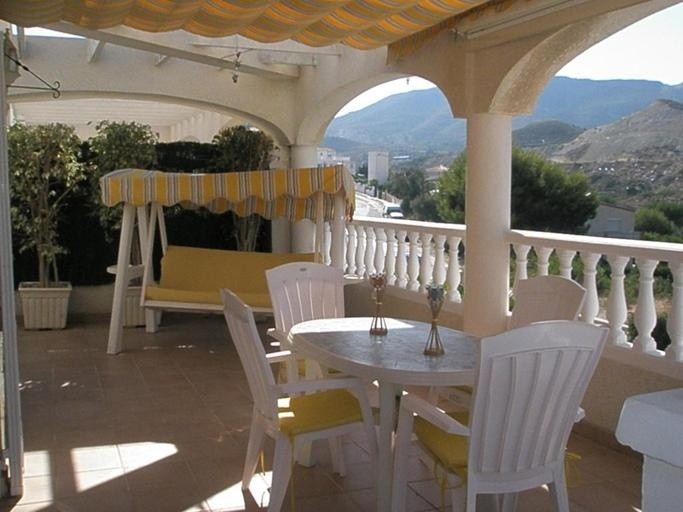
[614,386,683,512]
[288,317,492,511]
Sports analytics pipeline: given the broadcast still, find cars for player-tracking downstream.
[382,204,404,218]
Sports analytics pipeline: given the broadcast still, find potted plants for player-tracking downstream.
[206,125,275,324]
[4,121,90,330]
[89,119,163,327]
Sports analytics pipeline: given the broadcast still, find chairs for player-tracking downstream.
[401,276,586,424]
[219,287,379,512]
[389,319,610,512]
[263,262,380,477]
